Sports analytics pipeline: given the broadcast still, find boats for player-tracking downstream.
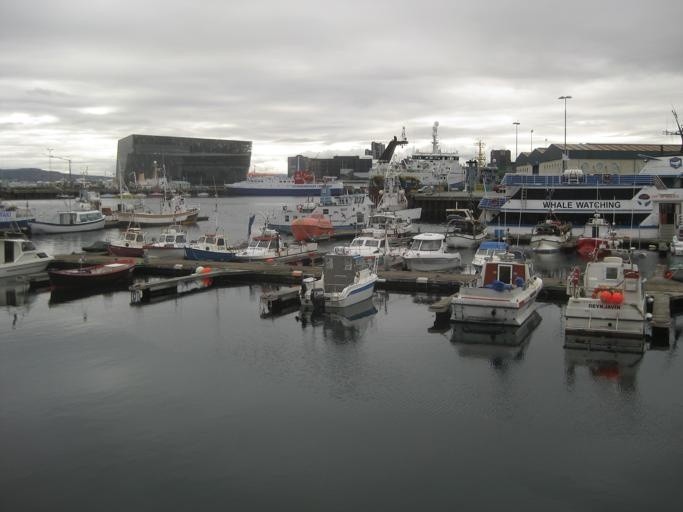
[562,170,656,356]
[450,183,545,326]
[299,200,388,310]
[365,135,487,224]
[223,157,345,197]
[472,165,683,250]
[260,175,376,241]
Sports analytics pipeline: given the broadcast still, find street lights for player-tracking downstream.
[512,121,521,161]
[556,95,573,147]
